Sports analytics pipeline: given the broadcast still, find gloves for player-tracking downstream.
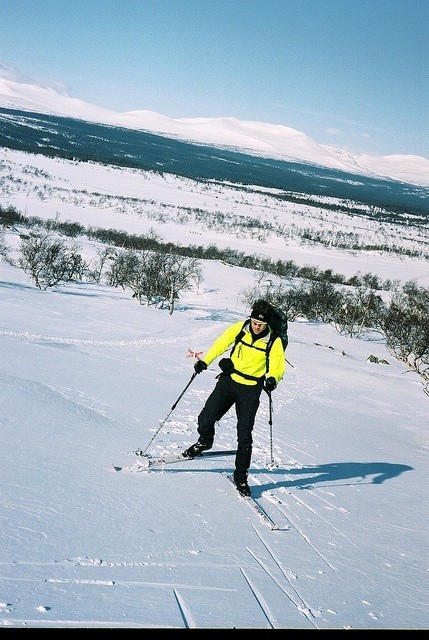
[194,360,206,373]
[266,377,277,391]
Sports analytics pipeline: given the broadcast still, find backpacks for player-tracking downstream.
[252,299,288,354]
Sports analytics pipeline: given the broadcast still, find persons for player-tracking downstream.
[180,307,287,497]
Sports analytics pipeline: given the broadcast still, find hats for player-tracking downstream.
[250,309,271,322]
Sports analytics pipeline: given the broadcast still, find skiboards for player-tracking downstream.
[110,449,279,531]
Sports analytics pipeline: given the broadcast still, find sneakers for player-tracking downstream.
[187,439,212,457]
[234,476,251,496]
